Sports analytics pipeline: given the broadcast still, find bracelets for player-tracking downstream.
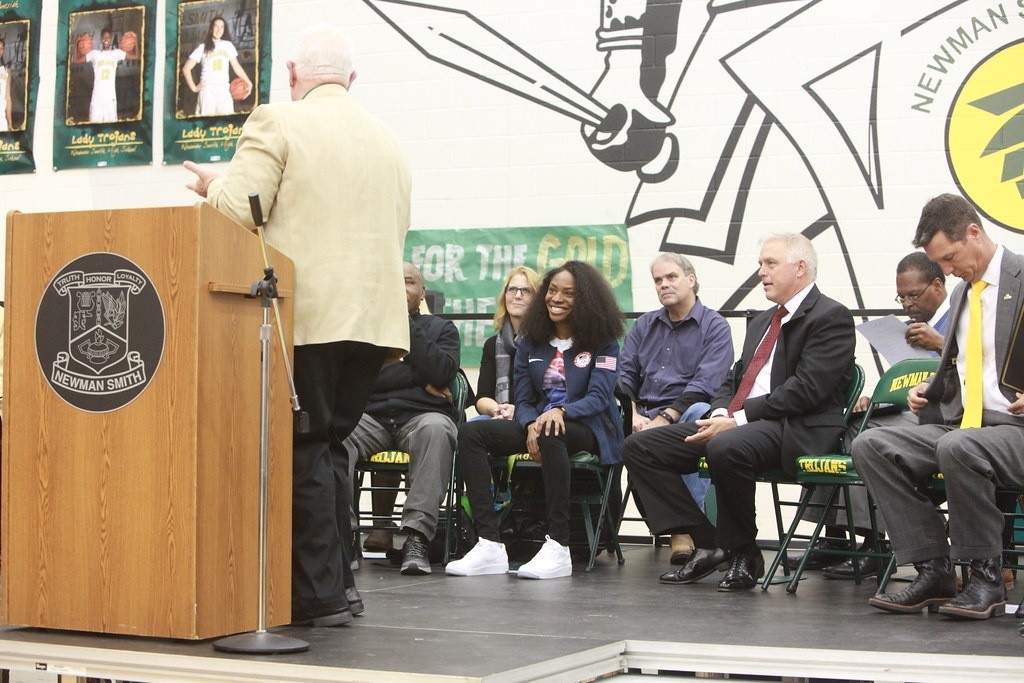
[559,407,565,412]
[659,411,674,424]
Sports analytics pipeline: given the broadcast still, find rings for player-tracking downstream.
[912,336,914,339]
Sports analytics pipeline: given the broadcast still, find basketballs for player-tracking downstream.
[78,38,93,55]
[230,78,250,100]
[120,31,137,52]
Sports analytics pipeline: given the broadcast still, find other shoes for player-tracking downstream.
[364,521,398,551]
[957,565,1015,592]
[670,532,695,563]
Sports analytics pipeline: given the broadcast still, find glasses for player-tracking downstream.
[505,286,535,296]
[895,276,942,305]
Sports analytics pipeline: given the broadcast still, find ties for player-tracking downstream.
[728,305,789,419]
[959,279,988,429]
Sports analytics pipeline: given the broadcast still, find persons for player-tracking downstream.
[445,260,623,580]
[182,17,253,114]
[619,252,734,561]
[342,261,460,574]
[75,28,139,122]
[851,193,1023,619]
[0,40,14,131]
[184,25,410,627]
[623,233,857,592]
[468,266,541,423]
[780,252,953,579]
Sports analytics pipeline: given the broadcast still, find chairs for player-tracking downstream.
[352,359,1022,593]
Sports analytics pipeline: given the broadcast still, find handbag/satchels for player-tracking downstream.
[387,503,478,563]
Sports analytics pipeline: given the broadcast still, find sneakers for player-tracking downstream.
[400,529,431,574]
[517,534,572,579]
[445,536,509,575]
[349,549,359,570]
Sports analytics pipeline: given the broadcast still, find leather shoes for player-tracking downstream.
[823,541,897,579]
[868,556,958,613]
[716,545,764,592]
[291,606,354,627]
[658,547,732,584]
[343,585,364,614]
[938,554,1008,620]
[778,535,849,570]
